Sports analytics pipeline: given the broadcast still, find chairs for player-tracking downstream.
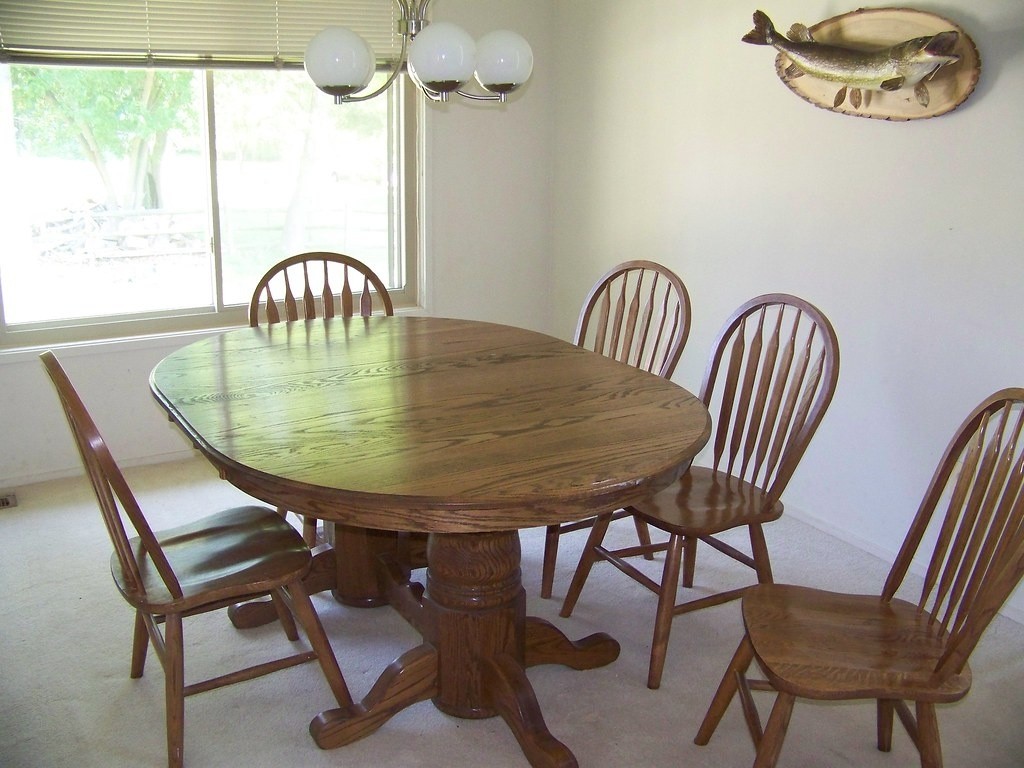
[694,386,1024,768]
[41,350,353,768]
[556,293,841,689]
[250,250,396,548]
[540,260,692,600]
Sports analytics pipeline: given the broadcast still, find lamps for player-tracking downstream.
[304,0,535,103]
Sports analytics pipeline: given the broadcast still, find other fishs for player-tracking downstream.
[741,8,963,109]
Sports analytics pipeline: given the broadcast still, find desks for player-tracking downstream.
[147,315,715,768]
